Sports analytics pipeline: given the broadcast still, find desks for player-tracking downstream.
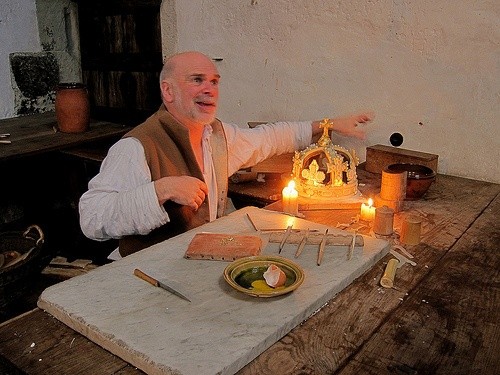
[0,111,130,163]
[0,162,500,375]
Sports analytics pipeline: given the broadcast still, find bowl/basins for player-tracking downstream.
[389,163,435,202]
[224,255,305,298]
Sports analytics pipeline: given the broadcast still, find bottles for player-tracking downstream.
[57,83,89,134]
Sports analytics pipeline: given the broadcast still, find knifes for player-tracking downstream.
[134,269,192,306]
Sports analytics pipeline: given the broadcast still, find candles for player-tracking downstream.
[281,181,299,215]
[361,198,376,220]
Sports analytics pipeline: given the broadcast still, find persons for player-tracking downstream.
[79,50,376,261]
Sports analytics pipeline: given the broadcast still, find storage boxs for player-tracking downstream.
[365,143,439,175]
